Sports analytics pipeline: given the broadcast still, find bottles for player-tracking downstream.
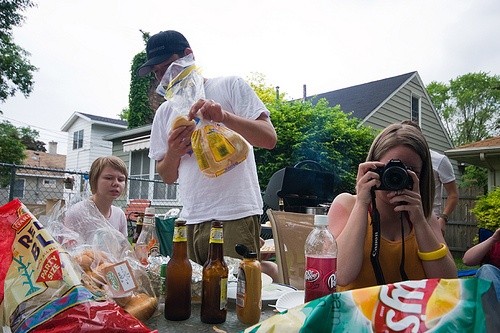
[235,244,262,324]
[165,220,191,321]
[200,221,228,324]
[136,242,148,264]
[134,208,161,258]
[304,215,337,302]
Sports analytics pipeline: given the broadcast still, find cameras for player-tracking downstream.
[367,159,414,191]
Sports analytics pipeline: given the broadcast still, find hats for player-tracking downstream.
[138,31,191,77]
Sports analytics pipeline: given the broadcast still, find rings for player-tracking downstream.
[210,100,215,104]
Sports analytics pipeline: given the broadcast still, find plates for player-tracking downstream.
[227,282,298,305]
[261,272,273,289]
[275,291,304,312]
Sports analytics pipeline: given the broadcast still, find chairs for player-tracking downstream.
[155,217,176,258]
[268,208,314,290]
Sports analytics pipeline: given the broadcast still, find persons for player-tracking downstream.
[401,120,459,234]
[463,228,500,268]
[138,30,277,265]
[327,125,457,292]
[62,156,131,260]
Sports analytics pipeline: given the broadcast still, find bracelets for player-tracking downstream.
[418,243,447,260]
[441,215,448,222]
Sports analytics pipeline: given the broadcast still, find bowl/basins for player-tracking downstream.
[305,207,324,215]
